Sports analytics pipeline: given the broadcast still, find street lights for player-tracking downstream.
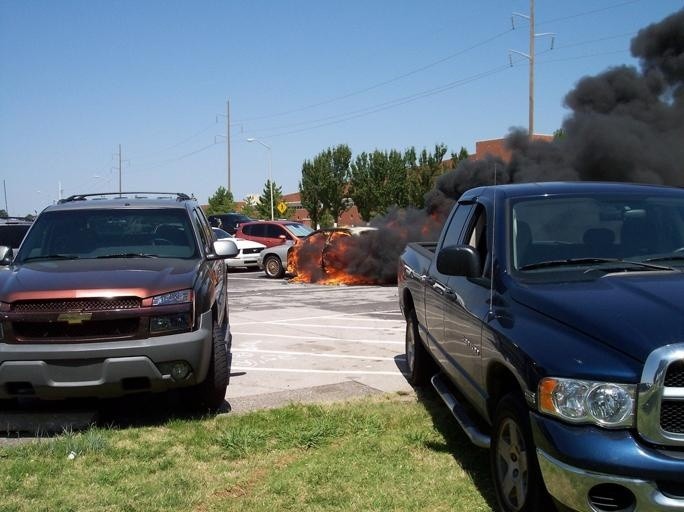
[247,138,274,222]
[95,175,121,196]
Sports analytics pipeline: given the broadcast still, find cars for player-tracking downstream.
[206,213,316,279]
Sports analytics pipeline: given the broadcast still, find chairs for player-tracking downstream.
[485,221,532,276]
[583,216,655,260]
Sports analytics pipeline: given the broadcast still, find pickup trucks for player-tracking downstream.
[397,180,684,511]
